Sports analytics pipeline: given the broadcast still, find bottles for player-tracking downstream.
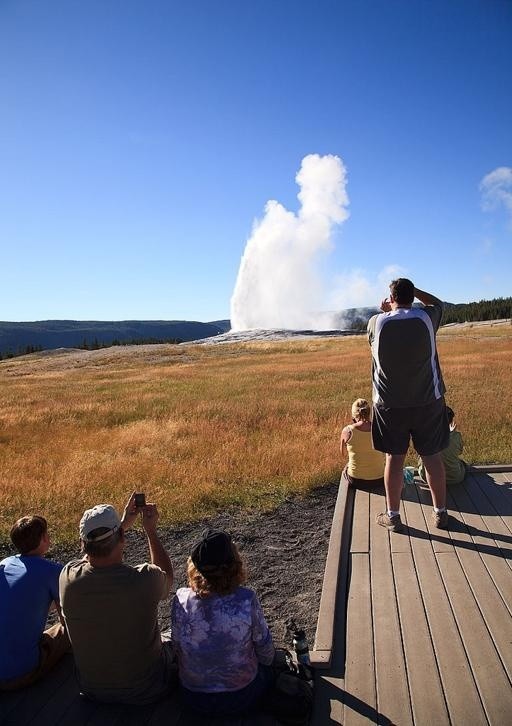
[293,629,311,665]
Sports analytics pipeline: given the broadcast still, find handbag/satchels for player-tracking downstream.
[255,647,316,710]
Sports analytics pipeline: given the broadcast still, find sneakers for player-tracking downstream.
[431,508,448,529]
[376,512,402,532]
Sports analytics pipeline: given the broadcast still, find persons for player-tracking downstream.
[1,516,65,693]
[58,491,175,705]
[367,278,456,531]
[339,400,385,490]
[172,530,289,714]
[416,407,469,485]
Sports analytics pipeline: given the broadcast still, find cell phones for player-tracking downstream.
[135,494,145,511]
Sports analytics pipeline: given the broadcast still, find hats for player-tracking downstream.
[80,504,121,543]
[191,527,231,571]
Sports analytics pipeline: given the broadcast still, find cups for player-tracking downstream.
[405,465,415,486]
